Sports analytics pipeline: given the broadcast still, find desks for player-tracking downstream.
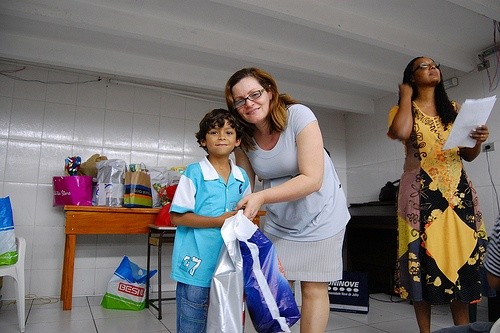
[60,205,266,310]
[145,224,178,321]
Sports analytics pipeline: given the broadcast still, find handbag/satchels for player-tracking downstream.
[0,196,18,266]
[234,209,301,333]
[53,153,156,209]
[99,256,157,311]
[205,208,244,333]
[378,178,401,207]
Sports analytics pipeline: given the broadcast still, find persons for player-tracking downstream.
[168,110,255,333]
[225,66,351,333]
[387,56,500,333]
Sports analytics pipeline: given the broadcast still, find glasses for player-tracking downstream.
[413,62,440,73]
[233,87,268,109]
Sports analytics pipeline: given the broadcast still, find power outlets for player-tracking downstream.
[482,143,494,152]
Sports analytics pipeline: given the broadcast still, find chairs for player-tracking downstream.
[0,237,26,333]
